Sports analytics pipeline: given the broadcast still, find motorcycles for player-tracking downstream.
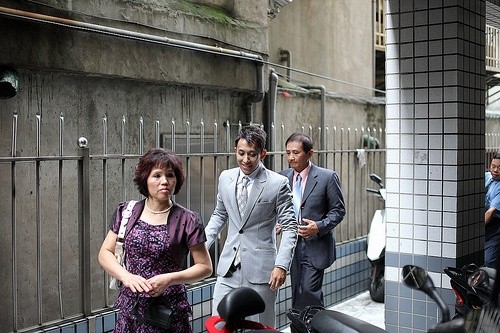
[365,173,386,302]
[204,263,500,333]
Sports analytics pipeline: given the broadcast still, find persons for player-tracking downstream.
[484,153,500,269]
[98,148,213,333]
[276,132,346,318]
[205,126,298,329]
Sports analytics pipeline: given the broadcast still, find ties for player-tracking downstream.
[485,178,494,194]
[238,175,250,220]
[293,175,302,225]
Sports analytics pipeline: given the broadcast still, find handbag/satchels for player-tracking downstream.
[132,293,174,330]
[109,200,138,290]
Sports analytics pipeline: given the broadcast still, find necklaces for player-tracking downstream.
[145,198,173,214]
